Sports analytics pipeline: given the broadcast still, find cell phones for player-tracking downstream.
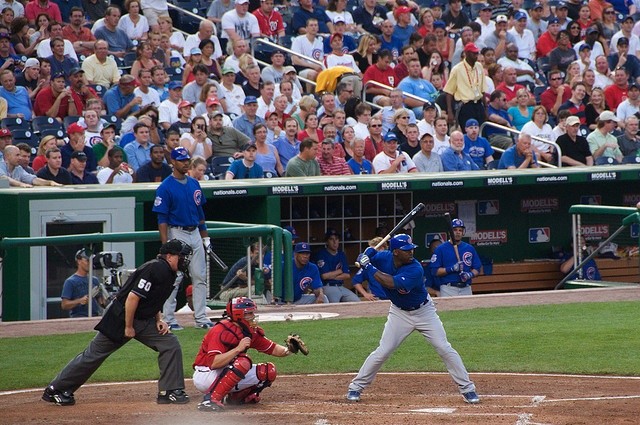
[198,124,203,130]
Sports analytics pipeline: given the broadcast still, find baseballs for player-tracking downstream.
[284,334,309,356]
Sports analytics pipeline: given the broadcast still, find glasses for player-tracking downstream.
[615,65,626,69]
[344,90,354,93]
[399,116,410,119]
[604,10,616,14]
[550,77,562,80]
[369,124,382,126]
[571,26,580,29]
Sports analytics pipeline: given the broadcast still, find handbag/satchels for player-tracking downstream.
[94,300,125,348]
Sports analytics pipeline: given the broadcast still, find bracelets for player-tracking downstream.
[530,161,535,165]
[366,263,378,275]
[446,267,452,274]
[468,272,474,278]
[198,223,207,231]
[67,99,74,103]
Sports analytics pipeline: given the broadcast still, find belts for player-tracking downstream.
[401,298,429,310]
[172,224,197,230]
[441,281,466,287]
[325,283,342,285]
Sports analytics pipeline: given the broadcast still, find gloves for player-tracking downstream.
[363,246,378,258]
[459,272,472,283]
[356,253,371,269]
[202,237,211,254]
[452,261,464,272]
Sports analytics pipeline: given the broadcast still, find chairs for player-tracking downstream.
[100,113,121,134]
[64,114,86,129]
[87,83,108,98]
[278,35,298,66]
[125,53,140,67]
[166,67,185,83]
[0,118,30,136]
[211,155,235,179]
[199,1,212,15]
[252,32,278,66]
[534,86,548,104]
[7,129,38,154]
[173,0,205,31]
[30,115,62,134]
[538,55,556,76]
[109,52,124,69]
[39,128,66,141]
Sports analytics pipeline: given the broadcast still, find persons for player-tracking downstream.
[102,74,142,117]
[610,14,640,59]
[253,123,284,178]
[34,71,78,117]
[315,230,360,303]
[393,45,423,81]
[480,47,496,67]
[119,115,152,148]
[92,122,128,166]
[451,25,474,68]
[291,17,324,94]
[487,0,515,23]
[219,242,266,300]
[529,0,557,21]
[556,116,594,167]
[352,0,388,34]
[553,109,582,140]
[573,28,605,61]
[84,0,108,21]
[582,69,595,104]
[170,101,194,137]
[433,20,455,62]
[0,144,63,188]
[45,36,80,88]
[262,50,304,94]
[417,7,436,37]
[347,139,373,174]
[599,5,620,44]
[180,115,213,160]
[586,110,623,166]
[485,14,515,59]
[460,51,466,60]
[397,122,421,159]
[588,0,613,20]
[485,89,513,150]
[606,0,636,22]
[264,110,286,145]
[224,142,263,180]
[553,2,573,30]
[162,129,180,163]
[417,33,437,69]
[421,49,448,92]
[497,133,538,169]
[365,117,384,162]
[151,66,169,102]
[124,122,155,172]
[69,151,99,184]
[592,55,615,91]
[518,104,554,162]
[373,88,416,136]
[322,32,362,76]
[0,128,12,157]
[353,102,372,140]
[91,15,106,35]
[559,234,601,281]
[571,44,595,73]
[15,57,45,99]
[184,19,223,60]
[140,0,169,31]
[589,21,609,56]
[602,67,629,111]
[61,249,111,318]
[586,86,610,132]
[265,95,291,130]
[200,97,234,128]
[550,30,578,83]
[285,137,320,177]
[57,109,103,148]
[317,138,351,176]
[317,124,346,160]
[507,88,534,131]
[527,2,547,43]
[431,72,444,93]
[377,20,403,64]
[0,8,14,29]
[429,1,442,21]
[341,125,355,162]
[563,62,580,87]
[512,0,530,20]
[443,0,468,33]
[182,64,219,108]
[152,146,215,331]
[566,0,582,21]
[221,0,261,56]
[185,158,207,180]
[158,80,197,130]
[97,148,136,184]
[292,0,335,35]
[192,296,309,411]
[363,49,400,107]
[536,18,572,57]
[412,132,444,172]
[497,42,541,94]
[347,233,481,403]
[41,238,194,406]
[439,130,480,171]
[219,67,246,117]
[59,122,97,172]
[390,107,410,144]
[182,47,202,85]
[374,36,382,54]
[223,39,257,73]
[67,67,105,116]
[0,0,25,18]
[384,4,418,26]
[386,0,420,19]
[198,39,223,84]
[81,39,121,89]
[607,37,640,85]
[0,69,36,122]
[416,101,437,137]
[453,22,487,65]
[429,218,482,297]
[432,116,450,154]
[134,69,161,107]
[256,79,275,119]
[273,118,301,170]
[317,93,336,123]
[30,12,50,49]
[424,239,443,297]
[85,98,108,128]
[53,0,90,28]
[333,109,346,143]
[38,58,52,85]
[0,24,16,54]
[557,82,591,138]
[36,148,72,185]
[252,0,286,38]
[94,6,134,58]
[32,134,57,173]
[275,65,302,103]
[266,242,329,305]
[25,0,62,25]
[376,226,389,238]
[279,80,301,117]
[324,0,366,34]
[137,145,172,182]
[298,113,326,143]
[352,34,379,74]
[576,3,592,41]
[540,71,571,129]
[477,54,489,76]
[159,34,186,69]
[616,113,640,156]
[119,104,161,144]
[206,110,252,162]
[242,63,264,98]
[324,18,357,56]
[508,7,519,29]
[292,96,333,133]
[148,30,165,67]
[352,237,390,302]
[196,82,228,116]
[0,33,26,74]
[263,225,300,297]
[474,4,496,40]
[508,12,538,61]
[206,0,235,36]
[118,0,149,40]
[37,21,79,62]
[372,131,417,174]
[16,142,34,175]
[317,81,354,118]
[11,16,46,56]
[616,81,640,133]
[63,6,97,54]
[131,42,162,78]
[0,97,7,120]
[567,21,581,49]
[157,15,185,55]
[232,95,268,140]
[409,32,423,50]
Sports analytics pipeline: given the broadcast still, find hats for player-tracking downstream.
[271,48,288,65]
[566,116,580,126]
[599,109,624,122]
[496,14,507,24]
[418,6,433,21]
[285,226,300,238]
[70,66,85,76]
[67,121,88,133]
[209,110,223,119]
[618,36,629,45]
[579,43,591,51]
[531,3,544,10]
[119,75,141,87]
[383,131,398,141]
[282,65,297,75]
[464,41,479,53]
[628,81,640,90]
[466,118,479,126]
[514,11,527,20]
[0,128,12,137]
[0,31,13,40]
[556,1,569,10]
[480,2,494,10]
[100,121,116,133]
[546,18,560,29]
[242,141,257,150]
[71,150,88,161]
[168,80,183,89]
[423,102,436,110]
[332,16,346,24]
[330,32,342,43]
[451,218,466,228]
[178,100,194,109]
[235,0,250,4]
[393,5,413,18]
[433,20,448,37]
[190,47,202,54]
[50,71,66,80]
[22,57,40,73]
[222,66,236,75]
[171,146,191,159]
[417,130,434,141]
[390,233,420,250]
[586,26,599,37]
[265,110,278,123]
[206,95,221,106]
[244,95,257,103]
[622,14,635,23]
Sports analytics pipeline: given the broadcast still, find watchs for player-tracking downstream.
[79,41,82,47]
[464,118,495,167]
[616,66,620,70]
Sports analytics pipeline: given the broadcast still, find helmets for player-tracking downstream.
[224,297,258,333]
[160,238,193,279]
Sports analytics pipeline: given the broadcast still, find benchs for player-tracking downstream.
[236,251,639,297]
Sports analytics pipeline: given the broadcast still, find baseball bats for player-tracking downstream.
[444,212,463,273]
[355,203,425,268]
[204,244,228,272]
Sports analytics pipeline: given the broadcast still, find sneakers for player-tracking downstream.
[41,387,76,405]
[226,394,261,404]
[347,391,360,402]
[295,242,311,252]
[157,389,189,404]
[169,324,184,330]
[461,392,480,404]
[197,398,227,413]
[194,321,216,328]
